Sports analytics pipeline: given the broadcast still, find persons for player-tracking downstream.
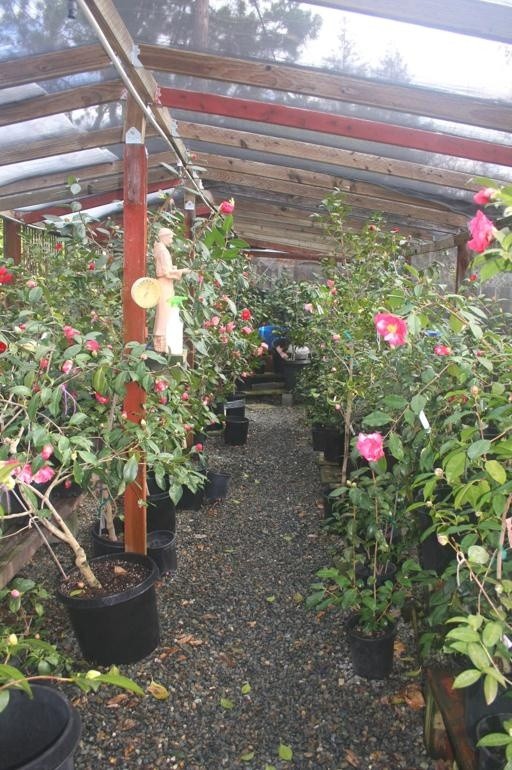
[152,227,191,355]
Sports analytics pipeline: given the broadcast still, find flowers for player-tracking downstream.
[0,578,144,716]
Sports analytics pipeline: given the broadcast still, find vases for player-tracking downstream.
[57,552,160,668]
[0,682,82,770]
